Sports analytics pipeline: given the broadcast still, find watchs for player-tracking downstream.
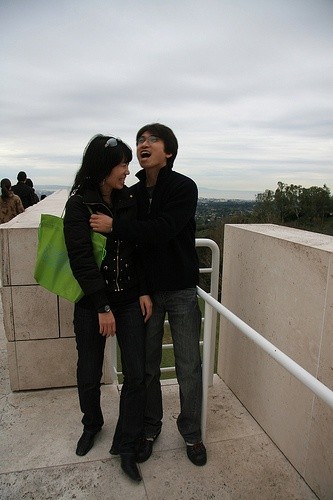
[97,305,110,313]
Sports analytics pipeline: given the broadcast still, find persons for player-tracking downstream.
[89,123,208,467]
[0,171,47,224]
[62,135,153,482]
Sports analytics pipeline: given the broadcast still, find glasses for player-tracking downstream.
[104,137,118,148]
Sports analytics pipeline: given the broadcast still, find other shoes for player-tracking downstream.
[75,425,103,456]
[118,429,162,484]
[185,430,207,467]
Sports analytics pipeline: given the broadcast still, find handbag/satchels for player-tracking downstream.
[33,193,106,304]
